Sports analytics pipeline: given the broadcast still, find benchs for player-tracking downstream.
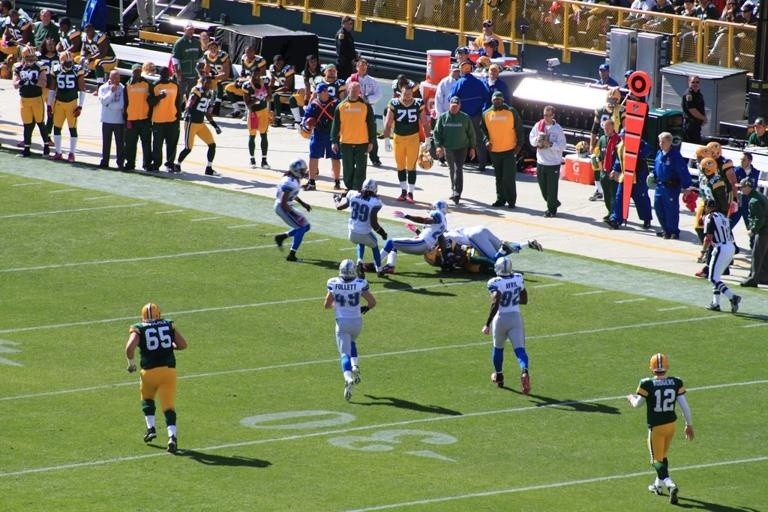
[219,64,309,129]
[107,43,172,86]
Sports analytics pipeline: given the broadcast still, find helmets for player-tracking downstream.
[683,141,724,212]
[140,301,160,321]
[434,199,450,214]
[362,179,378,194]
[426,248,440,264]
[339,257,356,278]
[494,257,512,276]
[650,353,670,375]
[289,158,308,177]
[20,46,74,64]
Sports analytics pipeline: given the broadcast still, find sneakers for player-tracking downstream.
[731,296,740,314]
[590,189,652,228]
[707,301,721,311]
[250,161,273,170]
[166,438,178,452]
[502,239,543,256]
[16,140,75,163]
[143,429,158,442]
[274,233,304,263]
[343,369,360,401]
[656,227,679,239]
[492,198,519,209]
[521,371,530,393]
[449,193,465,204]
[696,252,762,287]
[99,158,222,177]
[491,372,504,387]
[544,206,560,218]
[357,260,396,279]
[305,179,417,204]
[649,479,678,505]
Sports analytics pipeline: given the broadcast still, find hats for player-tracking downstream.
[446,92,508,104]
[131,64,174,75]
[735,177,755,187]
[457,19,502,73]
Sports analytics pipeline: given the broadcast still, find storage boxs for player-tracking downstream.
[565,152,597,185]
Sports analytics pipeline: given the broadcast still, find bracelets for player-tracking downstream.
[127,357,134,366]
[700,249,706,254]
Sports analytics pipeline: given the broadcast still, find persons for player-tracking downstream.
[626,352,696,504]
[479,255,531,396]
[695,196,741,314]
[271,0,767,289]
[323,258,377,402]
[0,0,270,177]
[124,302,187,453]
